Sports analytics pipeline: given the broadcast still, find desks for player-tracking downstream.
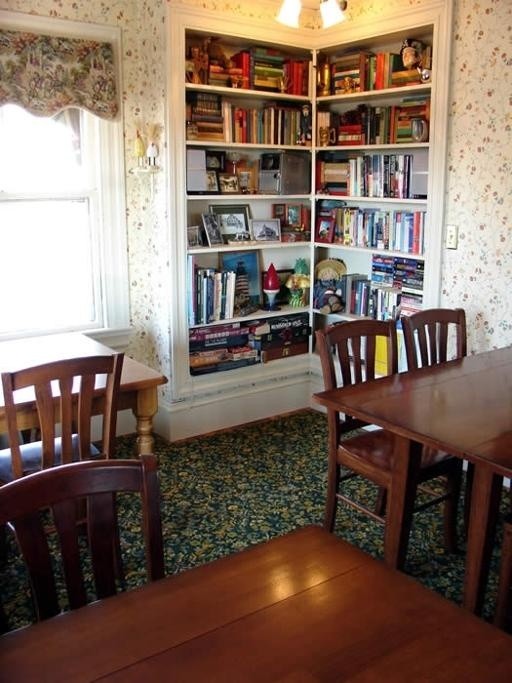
[313,346,511,630]
[2,330,169,454]
[1,525,511,683]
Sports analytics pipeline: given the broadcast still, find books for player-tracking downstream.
[316,97,430,146]
[189,315,312,375]
[316,150,413,199]
[186,90,311,146]
[340,255,425,328]
[335,333,401,374]
[279,153,310,194]
[316,47,430,95]
[274,202,310,241]
[334,207,426,254]
[187,254,236,325]
[186,36,313,97]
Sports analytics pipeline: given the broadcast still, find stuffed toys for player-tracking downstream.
[315,259,346,314]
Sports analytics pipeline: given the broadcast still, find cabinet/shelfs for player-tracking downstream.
[162,23,313,378]
[314,22,437,375]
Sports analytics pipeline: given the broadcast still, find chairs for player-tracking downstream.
[1,353,125,598]
[311,318,462,570]
[400,307,474,538]
[1,460,165,635]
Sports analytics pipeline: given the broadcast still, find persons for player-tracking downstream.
[400,39,427,69]
